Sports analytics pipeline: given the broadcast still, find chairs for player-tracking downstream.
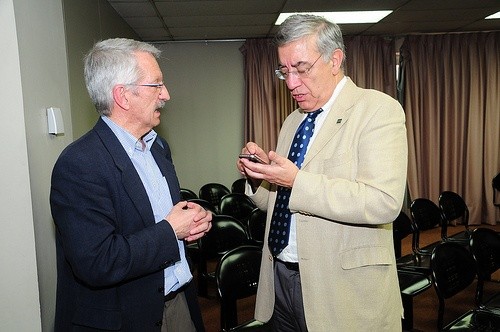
[179,179,500,332]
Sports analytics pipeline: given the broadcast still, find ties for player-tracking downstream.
[268,109,323,257]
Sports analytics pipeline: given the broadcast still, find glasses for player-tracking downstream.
[274,51,323,80]
[123,82,166,93]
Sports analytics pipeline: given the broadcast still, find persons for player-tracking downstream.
[236,14,408,332]
[48,38,213,332]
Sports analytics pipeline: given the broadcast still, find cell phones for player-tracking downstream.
[239,153,268,165]
